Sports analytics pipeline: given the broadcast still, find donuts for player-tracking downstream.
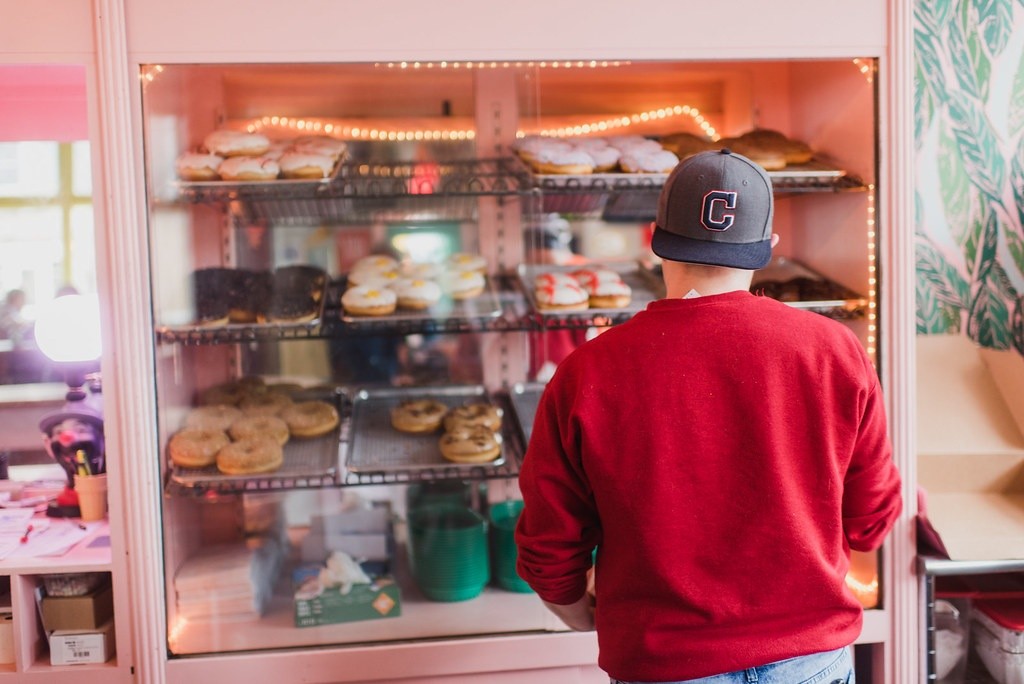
[165,372,506,475]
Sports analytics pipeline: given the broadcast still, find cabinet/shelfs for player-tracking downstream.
[914,332,1024,684]
[151,121,890,660]
[0,380,132,684]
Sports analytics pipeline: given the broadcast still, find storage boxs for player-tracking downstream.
[405,478,488,579]
[291,559,401,627]
[406,502,491,603]
[488,499,536,594]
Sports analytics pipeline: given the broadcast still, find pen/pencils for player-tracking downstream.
[74,521,87,530]
[77,450,92,477]
[21,524,34,543]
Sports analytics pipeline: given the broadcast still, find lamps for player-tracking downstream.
[34,293,103,401]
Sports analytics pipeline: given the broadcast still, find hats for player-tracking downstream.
[650,148,776,271]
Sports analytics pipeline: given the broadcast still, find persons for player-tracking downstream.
[510,151,905,682]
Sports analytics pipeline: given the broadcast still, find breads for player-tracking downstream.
[166,127,827,330]
